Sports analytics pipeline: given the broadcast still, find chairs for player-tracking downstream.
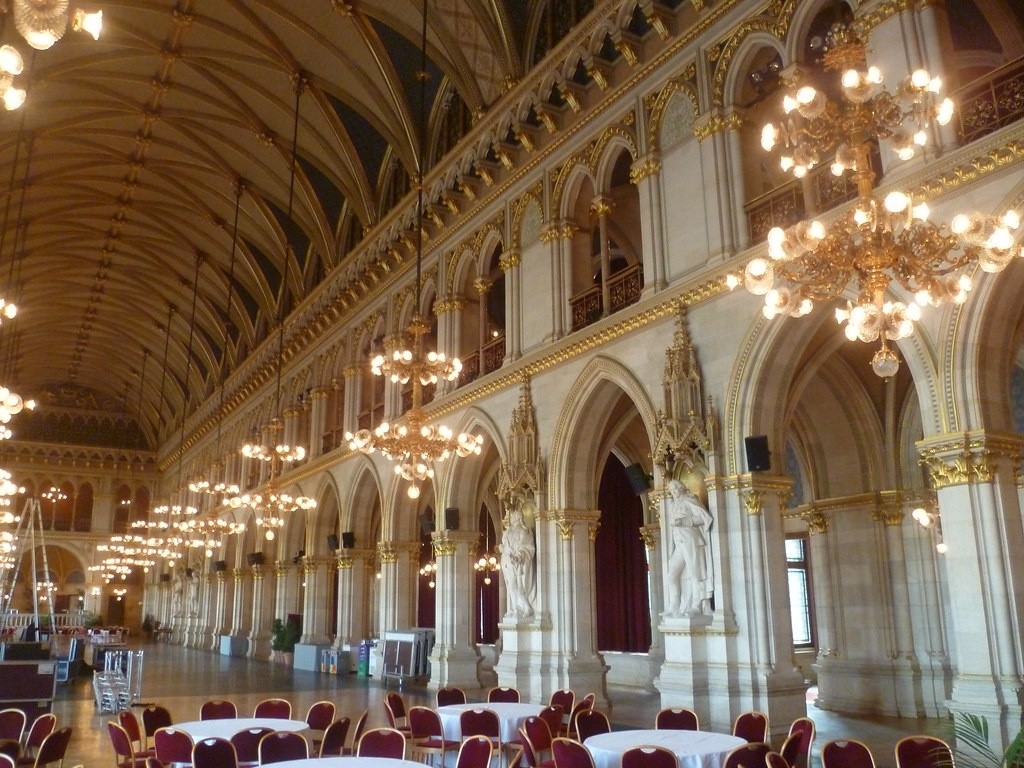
[0,686,958,768]
[0,622,131,647]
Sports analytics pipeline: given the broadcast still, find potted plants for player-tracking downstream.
[270,618,299,667]
[142,614,153,638]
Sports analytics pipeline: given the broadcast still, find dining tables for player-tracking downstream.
[582,728,749,768]
[163,716,315,768]
[433,700,556,768]
[256,756,434,768]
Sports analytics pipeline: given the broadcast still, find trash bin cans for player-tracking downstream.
[329,650,350,674]
[321,649,333,672]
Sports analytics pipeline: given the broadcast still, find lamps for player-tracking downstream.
[420,541,437,588]
[473,506,500,585]
[728,0,1024,380]
[0,2,311,616]
[344,0,488,499]
[913,500,946,555]
